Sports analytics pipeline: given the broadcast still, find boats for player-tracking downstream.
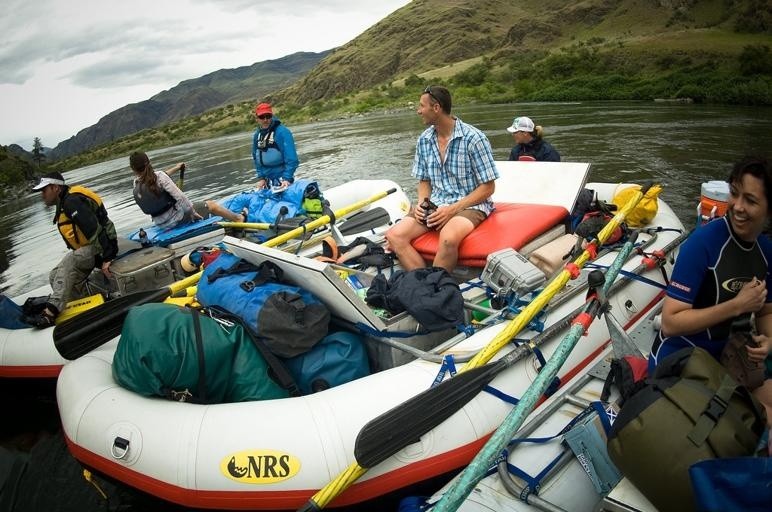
[0,179,411,378]
[55,181,689,512]
[416,302,658,512]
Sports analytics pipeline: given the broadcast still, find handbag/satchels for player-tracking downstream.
[365,267,464,331]
[113,254,373,401]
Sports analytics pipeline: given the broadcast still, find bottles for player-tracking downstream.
[138,228,149,248]
[421,196,436,229]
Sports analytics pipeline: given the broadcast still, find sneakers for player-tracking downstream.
[33,310,56,329]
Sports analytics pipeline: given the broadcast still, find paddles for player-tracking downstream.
[354,222,701,470]
[53,188,398,363]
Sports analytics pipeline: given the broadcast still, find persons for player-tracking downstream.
[248,101,303,207]
[125,149,248,235]
[505,115,563,165]
[32,170,122,333]
[382,81,503,279]
[658,149,772,459]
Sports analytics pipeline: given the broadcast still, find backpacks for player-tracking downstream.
[605,347,768,512]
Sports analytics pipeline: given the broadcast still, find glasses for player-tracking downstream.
[425,86,442,107]
[258,115,272,119]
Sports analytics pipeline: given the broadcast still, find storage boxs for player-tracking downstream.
[480,247,547,304]
[220,234,456,371]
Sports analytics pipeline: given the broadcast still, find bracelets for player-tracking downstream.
[190,210,197,216]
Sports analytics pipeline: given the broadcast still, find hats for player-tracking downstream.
[507,116,534,133]
[32,178,65,190]
[256,103,272,117]
